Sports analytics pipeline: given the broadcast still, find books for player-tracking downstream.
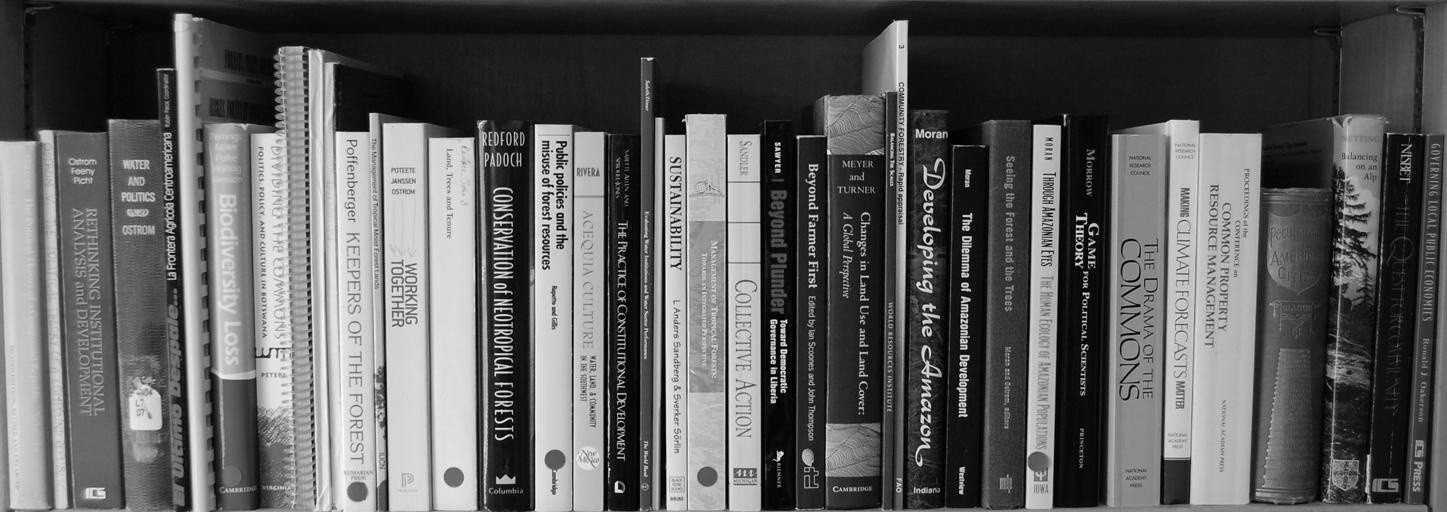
[2,8,1437,507]
